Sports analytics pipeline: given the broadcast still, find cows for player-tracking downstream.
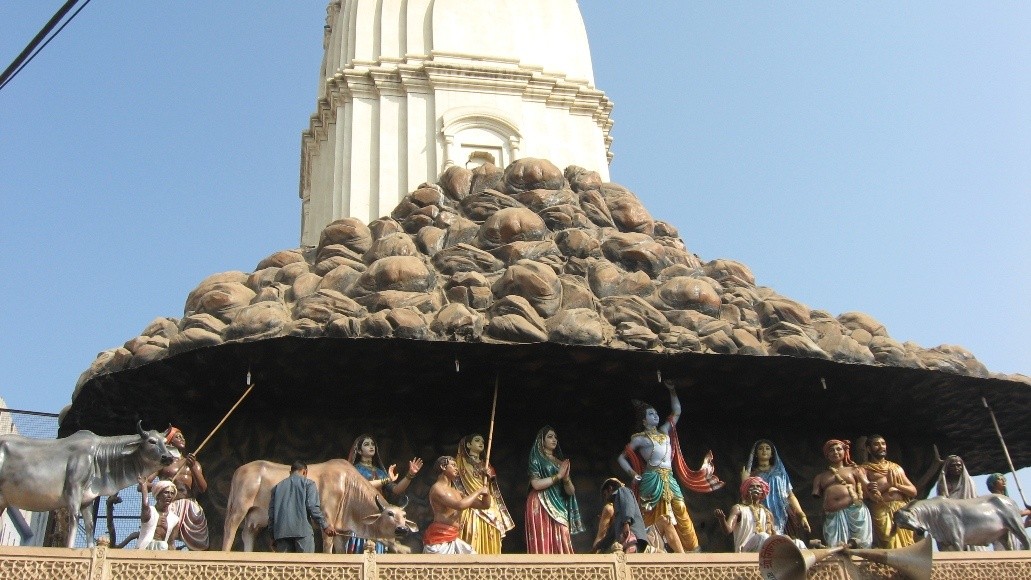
[892,493,1031,551]
[0,419,174,548]
[222,459,420,552]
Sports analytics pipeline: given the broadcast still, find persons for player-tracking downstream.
[934,455,987,551]
[423,432,515,554]
[135,426,207,550]
[593,477,685,553]
[813,433,918,550]
[985,472,1031,551]
[524,424,586,554]
[593,479,648,553]
[618,375,699,553]
[268,462,338,553]
[347,434,423,554]
[714,440,811,552]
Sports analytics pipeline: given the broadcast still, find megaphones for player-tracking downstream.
[759,535,861,580]
[846,533,932,580]
